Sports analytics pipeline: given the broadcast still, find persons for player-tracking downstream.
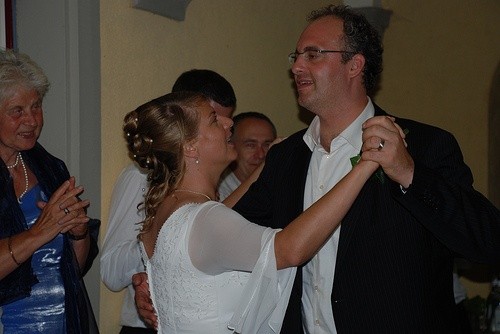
[217,112,277,203]
[122,92,408,334]
[132,4,500,334]
[100,69,237,334]
[0,49,101,334]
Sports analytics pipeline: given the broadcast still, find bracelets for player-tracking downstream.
[8,236,21,266]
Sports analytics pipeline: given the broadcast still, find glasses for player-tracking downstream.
[288,49,356,65]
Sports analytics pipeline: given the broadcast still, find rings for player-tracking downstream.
[377,138,385,151]
[63,208,70,214]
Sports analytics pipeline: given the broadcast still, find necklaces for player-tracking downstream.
[7,151,29,204]
[174,189,212,201]
